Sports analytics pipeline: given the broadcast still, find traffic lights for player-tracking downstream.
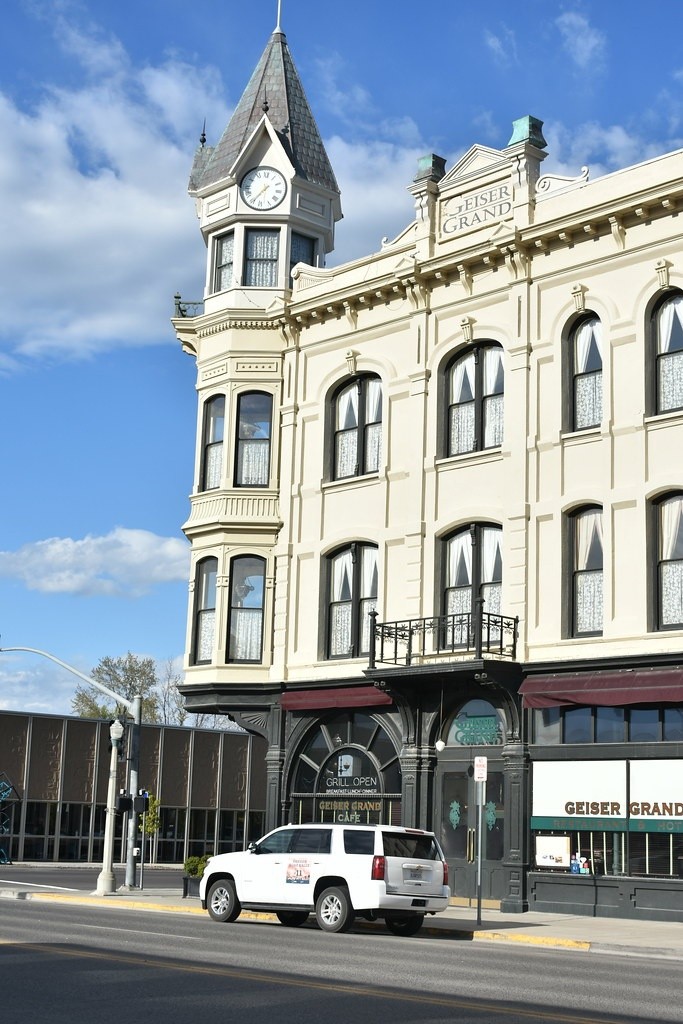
[134,797,149,813]
[108,715,127,756]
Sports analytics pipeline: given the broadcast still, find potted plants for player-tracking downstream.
[184,853,212,897]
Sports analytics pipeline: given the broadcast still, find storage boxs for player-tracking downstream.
[337,755,361,777]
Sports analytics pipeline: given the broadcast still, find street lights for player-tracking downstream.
[96,719,124,893]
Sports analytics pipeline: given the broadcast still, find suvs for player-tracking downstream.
[199,822,451,937]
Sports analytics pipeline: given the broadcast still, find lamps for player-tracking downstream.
[234,573,254,601]
[240,414,261,438]
[435,678,445,753]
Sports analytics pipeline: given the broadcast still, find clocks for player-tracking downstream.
[239,167,285,209]
